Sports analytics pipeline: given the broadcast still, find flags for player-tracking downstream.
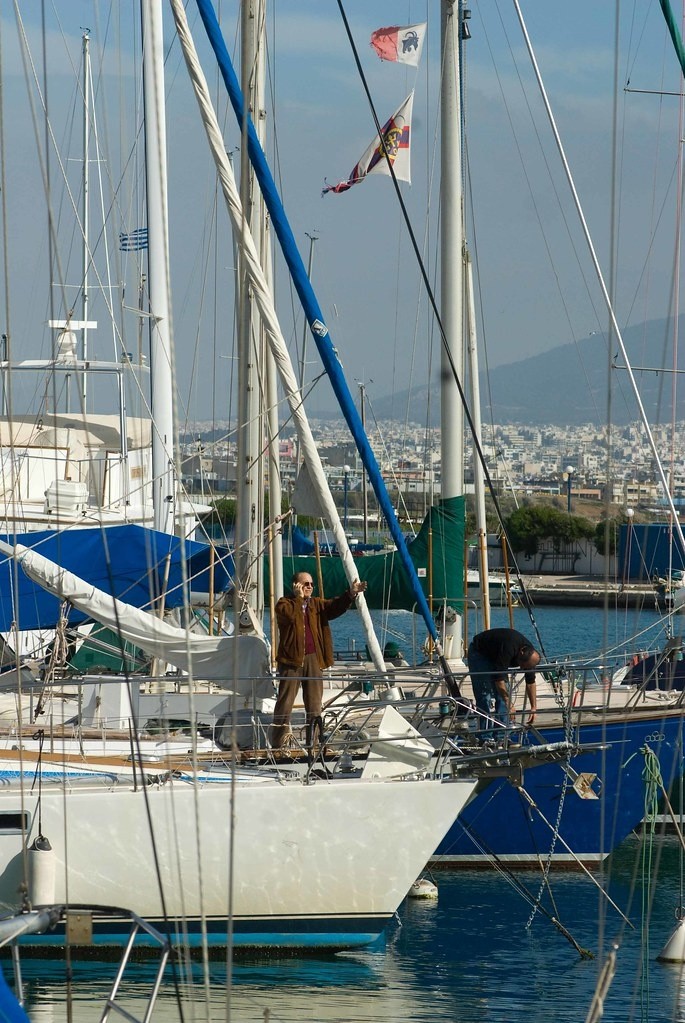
[320,90,414,199]
[370,21,425,67]
[119,228,148,252]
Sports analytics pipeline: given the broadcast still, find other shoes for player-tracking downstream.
[497,738,522,749]
[479,739,496,749]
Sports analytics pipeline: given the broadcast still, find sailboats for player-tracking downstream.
[0,0,685,1023]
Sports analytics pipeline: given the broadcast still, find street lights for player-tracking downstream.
[620,508,634,591]
[343,464,351,529]
[564,465,574,512]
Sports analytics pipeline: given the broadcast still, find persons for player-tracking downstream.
[273,571,367,763]
[467,628,541,746]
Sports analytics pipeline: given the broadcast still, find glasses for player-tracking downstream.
[304,582,314,587]
